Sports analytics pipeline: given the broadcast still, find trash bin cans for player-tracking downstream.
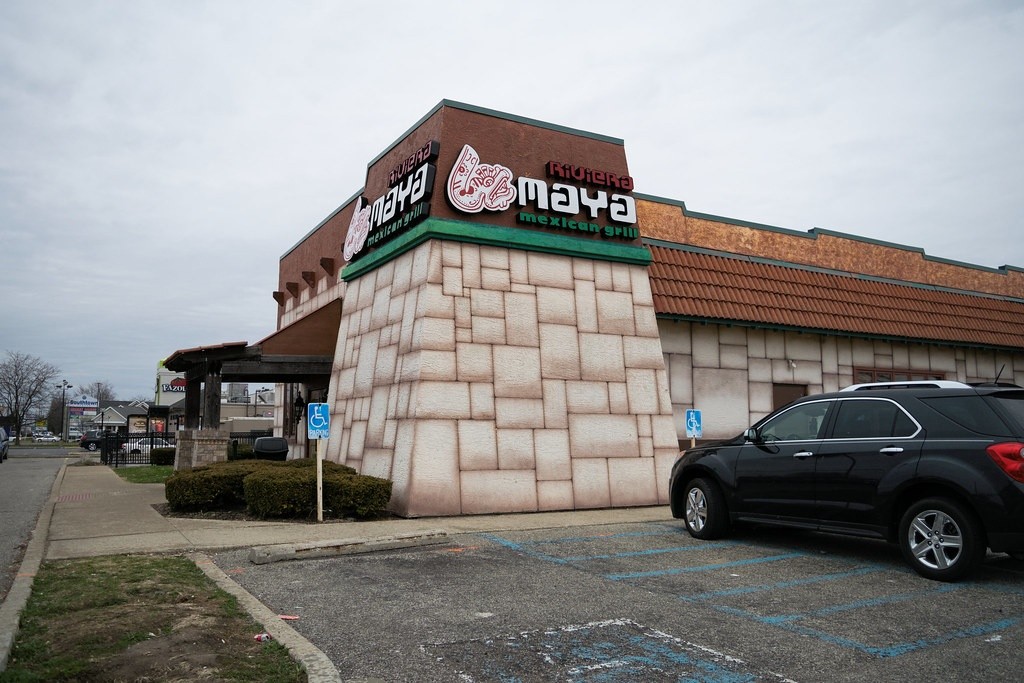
[253,436,290,462]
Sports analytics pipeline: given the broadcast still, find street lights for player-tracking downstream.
[56,380,73,441]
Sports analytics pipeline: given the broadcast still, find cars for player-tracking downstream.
[122,438,176,454]
[0,427,9,463]
[33,430,52,439]
[35,435,60,443]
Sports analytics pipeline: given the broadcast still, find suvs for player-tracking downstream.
[78,430,128,451]
[668,380,1024,584]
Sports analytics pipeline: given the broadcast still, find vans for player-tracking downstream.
[8,431,22,442]
